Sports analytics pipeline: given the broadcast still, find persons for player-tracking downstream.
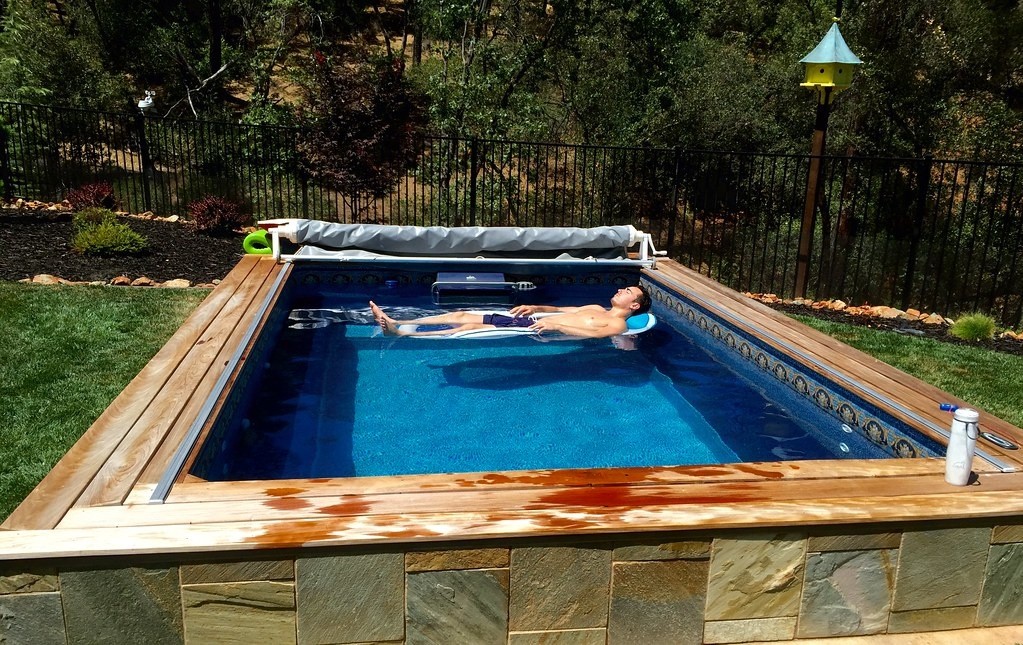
[369,285,652,338]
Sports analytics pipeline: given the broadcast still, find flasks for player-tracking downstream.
[945,406,980,486]
